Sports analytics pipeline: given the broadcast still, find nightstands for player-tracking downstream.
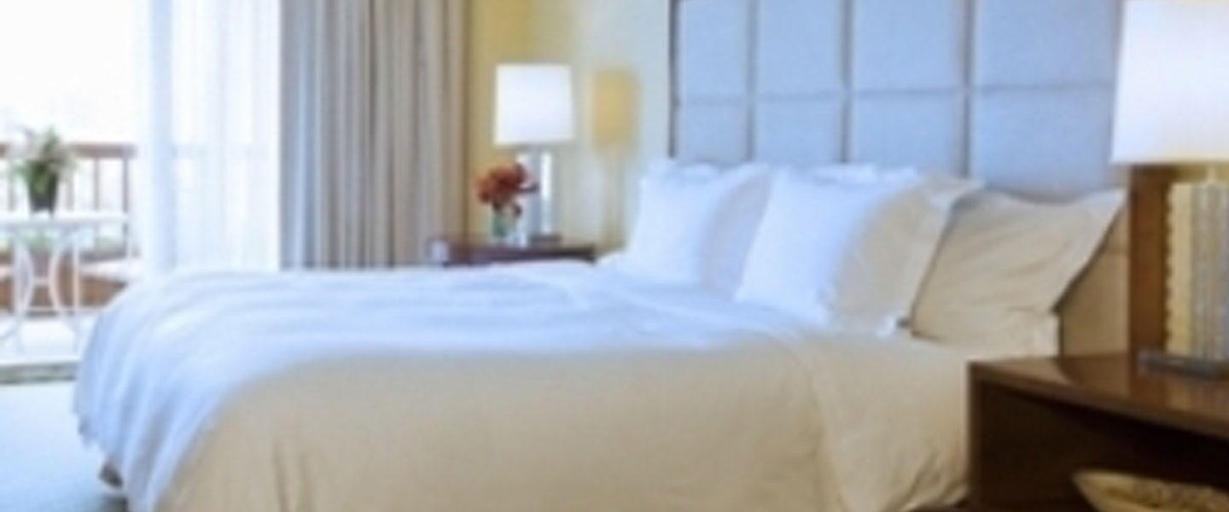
[442,222,598,286]
[962,353,1229,508]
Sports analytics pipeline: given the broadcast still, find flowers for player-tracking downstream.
[477,163,540,214]
[1,125,87,183]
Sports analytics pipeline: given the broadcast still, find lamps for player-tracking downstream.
[494,59,579,237]
[1108,2,1229,370]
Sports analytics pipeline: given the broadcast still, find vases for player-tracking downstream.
[23,165,61,212]
[483,198,518,234]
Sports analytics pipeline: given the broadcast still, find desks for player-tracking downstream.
[0,209,123,367]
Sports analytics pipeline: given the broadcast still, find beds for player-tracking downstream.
[77,238,1118,511]
